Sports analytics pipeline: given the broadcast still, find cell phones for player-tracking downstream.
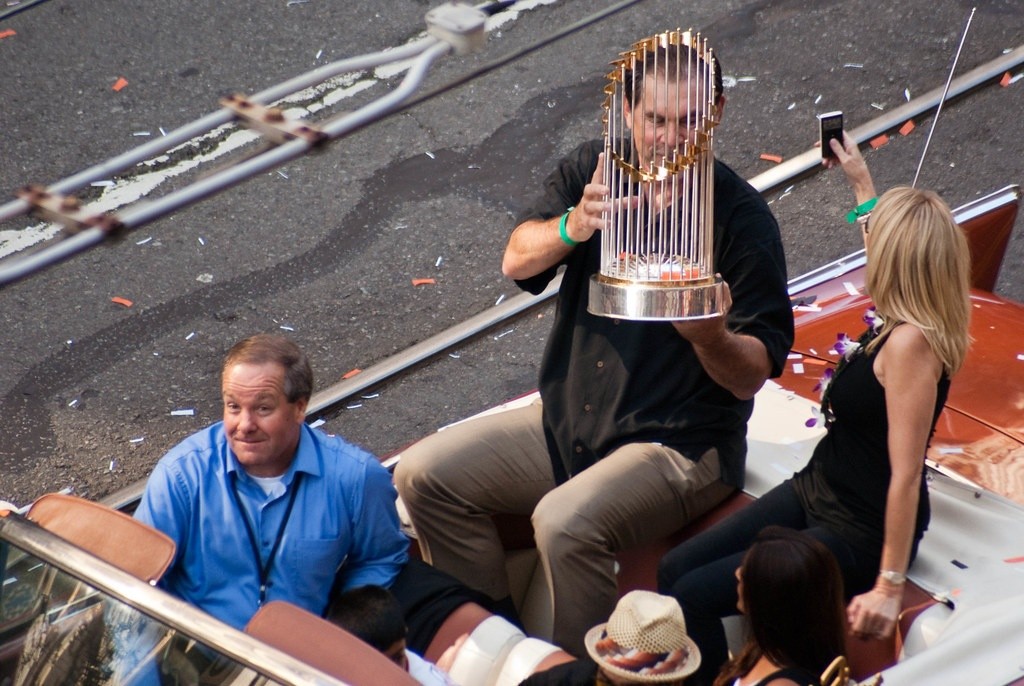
[818,110,844,158]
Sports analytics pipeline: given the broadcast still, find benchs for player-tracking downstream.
[618,492,931,681]
[386,556,581,686]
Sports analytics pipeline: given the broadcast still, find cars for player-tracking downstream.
[1,180,1024,686]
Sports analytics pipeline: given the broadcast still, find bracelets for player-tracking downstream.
[879,567,907,585]
[561,207,580,247]
[846,196,880,223]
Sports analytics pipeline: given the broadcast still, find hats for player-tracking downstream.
[583,591,704,684]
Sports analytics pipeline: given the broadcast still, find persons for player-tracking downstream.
[686,132,979,685]
[519,589,711,686]
[709,546,851,686]
[105,336,408,685]
[363,39,796,677]
